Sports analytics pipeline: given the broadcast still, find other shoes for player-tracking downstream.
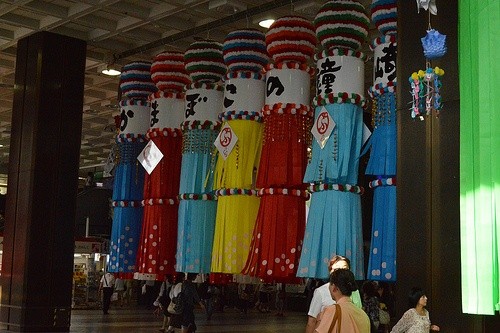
[103,308,109,315]
[159,328,175,333]
[119,303,129,307]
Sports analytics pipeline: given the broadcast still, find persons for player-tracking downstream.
[390,288,440,333]
[362,279,394,333]
[99,266,283,333]
[306,255,371,333]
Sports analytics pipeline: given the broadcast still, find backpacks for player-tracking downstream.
[172,292,186,313]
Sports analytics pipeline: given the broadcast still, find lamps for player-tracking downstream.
[101,55,123,76]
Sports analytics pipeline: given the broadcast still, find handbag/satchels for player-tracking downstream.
[378,308,390,325]
[167,300,176,314]
[158,296,171,308]
[110,287,114,296]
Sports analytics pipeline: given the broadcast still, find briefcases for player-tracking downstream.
[111,293,118,301]
